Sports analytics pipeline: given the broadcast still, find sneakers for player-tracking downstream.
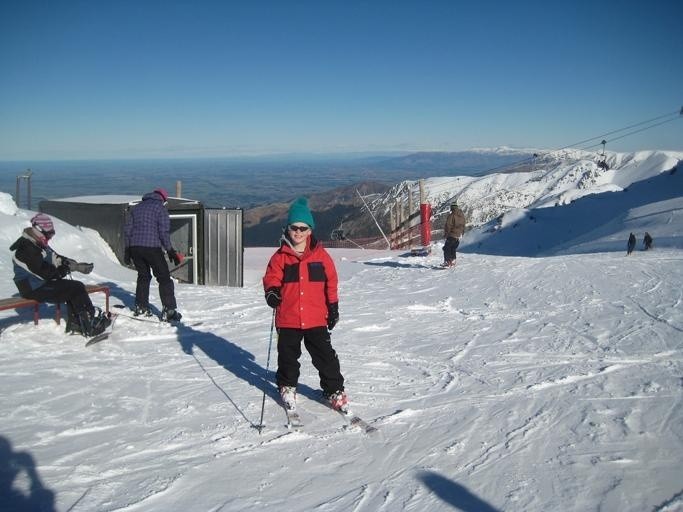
[135,303,152,316]
[279,385,296,406]
[440,260,451,267]
[162,310,182,321]
[328,391,349,410]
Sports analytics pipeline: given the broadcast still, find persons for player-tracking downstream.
[11,212,111,336]
[262,196,347,410]
[642,231,651,247]
[441,199,465,268]
[628,232,635,253]
[121,188,183,321]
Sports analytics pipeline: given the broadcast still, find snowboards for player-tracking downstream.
[111,305,201,327]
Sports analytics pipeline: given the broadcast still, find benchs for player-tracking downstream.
[1,285,111,327]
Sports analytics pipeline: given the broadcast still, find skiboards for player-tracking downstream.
[286,405,377,434]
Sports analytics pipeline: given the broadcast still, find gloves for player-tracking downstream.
[265,286,281,307]
[76,262,94,274]
[328,302,339,329]
[167,248,180,265]
[124,248,131,265]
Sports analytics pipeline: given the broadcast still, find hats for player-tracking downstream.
[154,188,168,201]
[288,197,313,227]
[31,214,53,232]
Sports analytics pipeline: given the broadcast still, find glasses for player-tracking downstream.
[33,224,55,239]
[290,224,308,232]
